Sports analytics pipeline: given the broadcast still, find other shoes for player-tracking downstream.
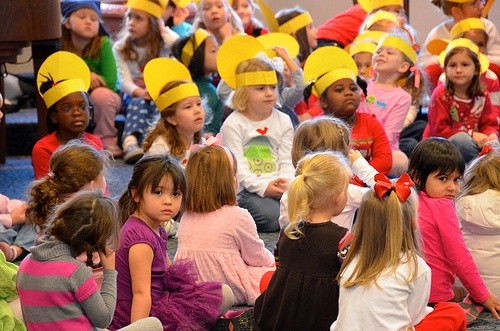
[457,289,486,326]
[1,240,13,261]
[103,142,122,157]
[122,144,145,164]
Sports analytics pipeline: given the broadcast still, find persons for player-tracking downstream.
[0,0,500,331]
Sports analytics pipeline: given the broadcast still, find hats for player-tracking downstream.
[58,1,111,39]
